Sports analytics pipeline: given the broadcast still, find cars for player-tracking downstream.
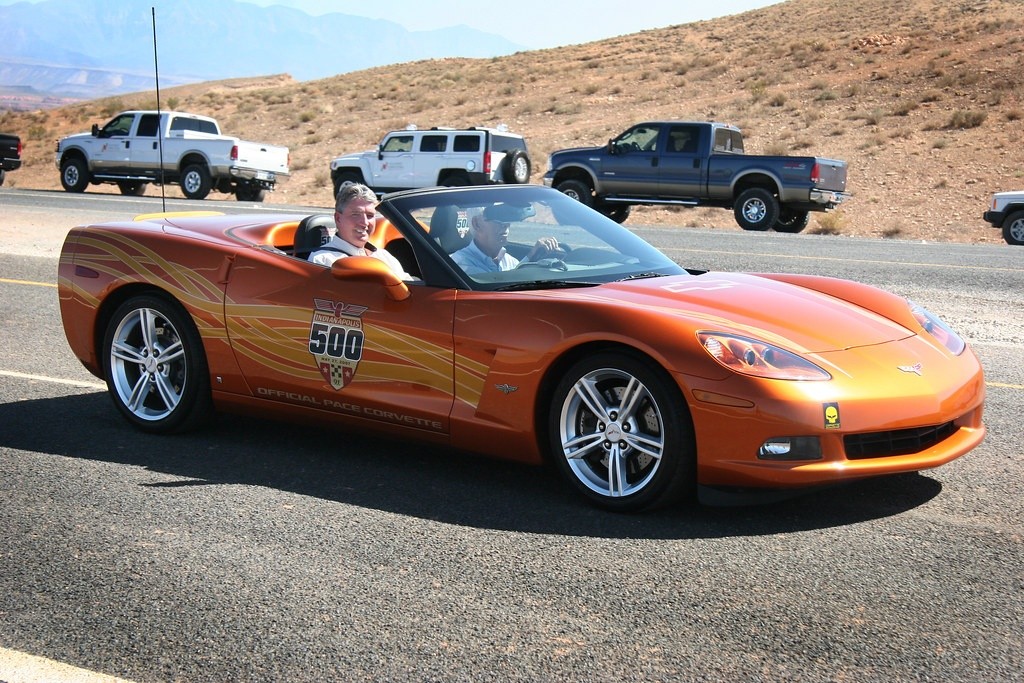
[984,185,1024,247]
[0,132,23,192]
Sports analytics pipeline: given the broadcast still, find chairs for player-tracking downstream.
[291,214,338,260]
[668,136,676,152]
[680,140,699,152]
[431,204,480,254]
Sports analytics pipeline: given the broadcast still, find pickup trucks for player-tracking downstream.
[53,110,291,202]
[543,122,847,233]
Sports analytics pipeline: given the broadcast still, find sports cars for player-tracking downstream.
[59,183,986,511]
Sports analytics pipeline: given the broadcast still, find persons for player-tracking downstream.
[449,203,560,276]
[307,181,414,281]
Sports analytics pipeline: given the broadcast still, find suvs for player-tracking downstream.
[330,124,532,206]
